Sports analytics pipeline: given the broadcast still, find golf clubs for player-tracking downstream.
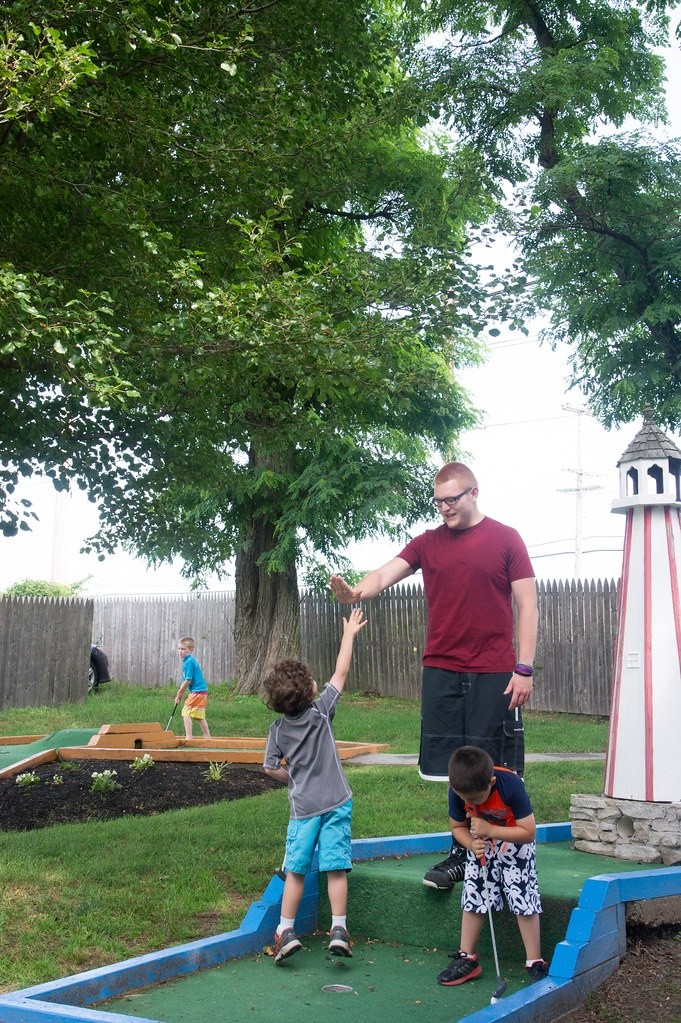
[165,703,179,731]
[478,836,507,998]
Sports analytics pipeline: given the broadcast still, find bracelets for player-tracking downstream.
[515,663,533,677]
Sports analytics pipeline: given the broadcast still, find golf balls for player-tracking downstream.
[491,995,503,1003]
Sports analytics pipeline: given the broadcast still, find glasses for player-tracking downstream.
[433,486,473,506]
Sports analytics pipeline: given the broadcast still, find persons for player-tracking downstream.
[174,637,211,740]
[261,608,369,963]
[435,745,549,986]
[329,462,539,890]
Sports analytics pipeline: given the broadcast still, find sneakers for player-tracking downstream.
[273,928,302,963]
[424,843,469,892]
[438,951,481,985]
[329,926,354,958]
[523,962,547,983]
